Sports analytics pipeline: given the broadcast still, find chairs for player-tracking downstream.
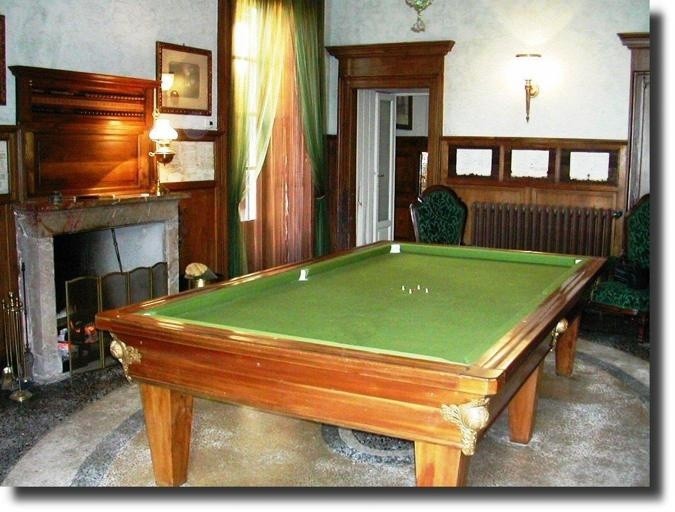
[409,185,468,244]
[583,193,650,344]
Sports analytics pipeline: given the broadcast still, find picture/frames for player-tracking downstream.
[395,95,412,129]
[0,125,17,205]
[157,42,212,116]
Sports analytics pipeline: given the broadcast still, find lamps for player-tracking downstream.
[148,118,178,197]
[515,54,541,121]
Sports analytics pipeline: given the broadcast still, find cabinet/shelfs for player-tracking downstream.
[616,34,650,213]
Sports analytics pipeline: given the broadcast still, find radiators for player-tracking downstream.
[470,200,622,258]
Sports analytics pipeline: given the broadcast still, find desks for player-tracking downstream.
[95,240,609,487]
